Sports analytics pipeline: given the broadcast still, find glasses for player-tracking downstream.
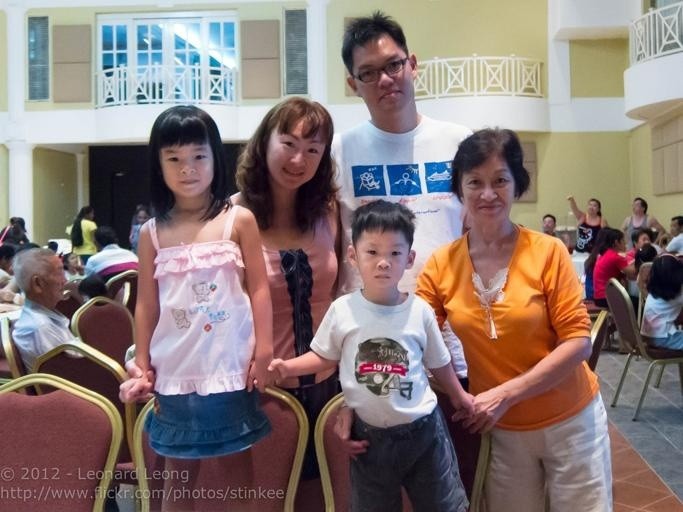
[351,54,409,83]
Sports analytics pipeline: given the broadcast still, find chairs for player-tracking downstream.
[584,310,607,371]
[0,244,488,512]
[602,278,682,422]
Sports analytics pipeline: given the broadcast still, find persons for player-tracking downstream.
[542,192,683,353]
[125,94,358,511]
[331,125,614,512]
[117,104,278,511]
[0,202,152,314]
[254,197,477,511]
[11,246,85,396]
[327,11,477,511]
